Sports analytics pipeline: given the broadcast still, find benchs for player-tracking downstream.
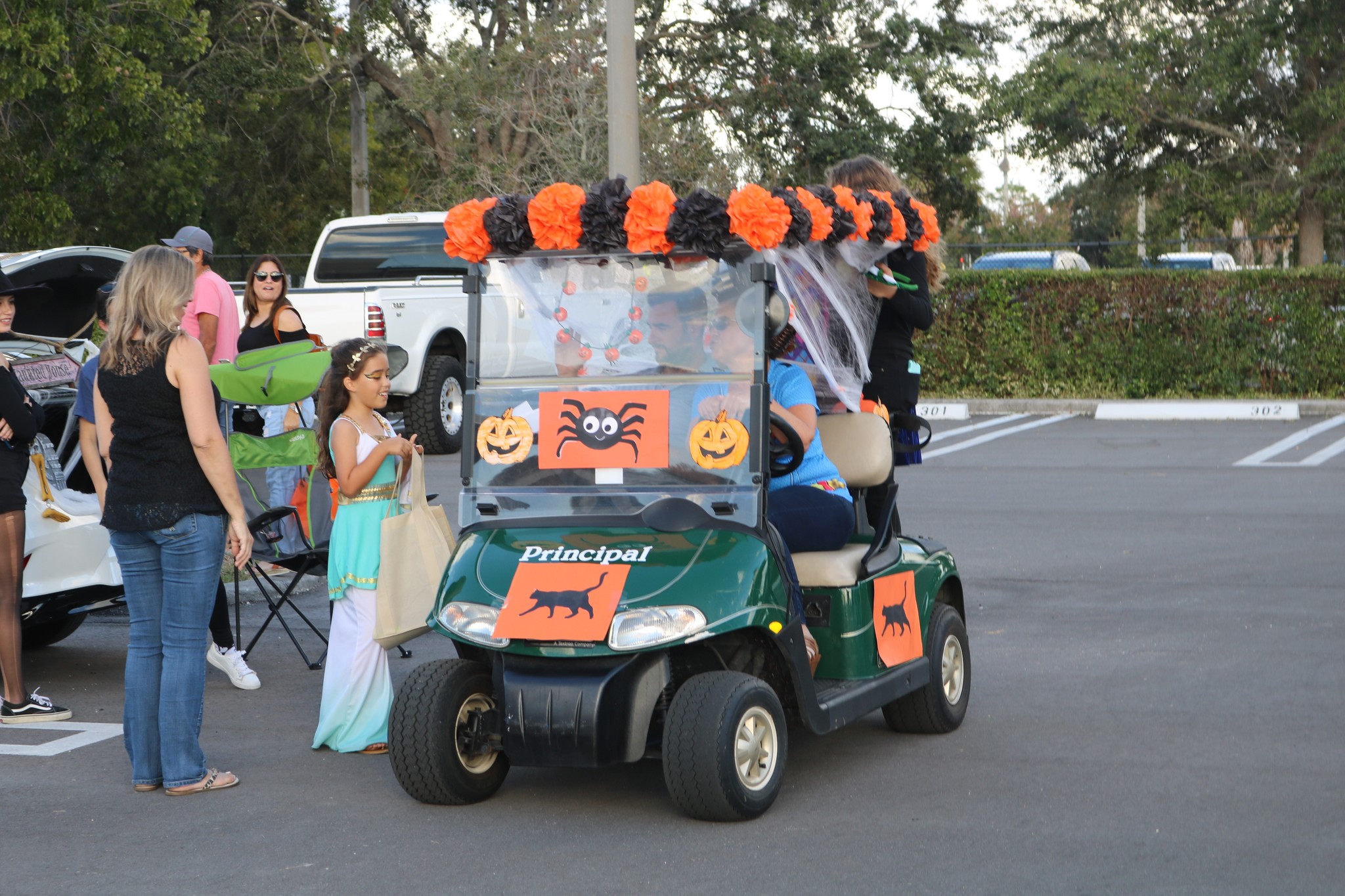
[788,412,893,588]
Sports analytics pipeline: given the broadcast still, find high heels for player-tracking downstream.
[805,636,822,678]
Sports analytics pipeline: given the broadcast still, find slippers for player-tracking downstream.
[165,768,239,796]
[134,782,162,791]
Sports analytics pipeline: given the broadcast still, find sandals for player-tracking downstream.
[359,743,388,754]
[246,564,290,576]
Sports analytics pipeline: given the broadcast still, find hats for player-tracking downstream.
[160,226,213,254]
[0,270,54,304]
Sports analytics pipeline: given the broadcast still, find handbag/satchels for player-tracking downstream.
[372,445,458,651]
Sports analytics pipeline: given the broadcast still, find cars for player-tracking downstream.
[0,243,233,651]
[967,250,1091,273]
[1141,250,1237,271]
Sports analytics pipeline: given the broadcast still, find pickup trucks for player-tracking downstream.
[218,208,526,456]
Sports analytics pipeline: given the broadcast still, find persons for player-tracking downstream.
[0,270,72,724]
[554,280,855,677]
[77,244,255,796]
[312,337,423,754]
[73,226,317,690]
[824,154,948,465]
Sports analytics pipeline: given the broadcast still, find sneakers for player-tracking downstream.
[206,641,261,690]
[0,687,72,724]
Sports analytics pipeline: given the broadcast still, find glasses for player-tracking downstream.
[708,315,736,331]
[255,271,284,282]
[97,282,116,294]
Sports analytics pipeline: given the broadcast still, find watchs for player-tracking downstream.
[288,405,301,414]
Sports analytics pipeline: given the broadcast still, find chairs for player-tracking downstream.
[206,339,440,671]
